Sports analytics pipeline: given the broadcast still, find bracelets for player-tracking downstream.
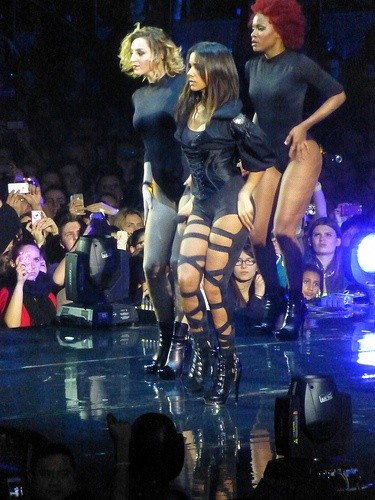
[313,183,322,191]
[143,181,151,187]
[255,293,262,298]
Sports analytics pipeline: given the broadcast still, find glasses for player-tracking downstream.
[136,241,144,246]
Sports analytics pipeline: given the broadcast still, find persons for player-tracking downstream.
[116,23,193,379]
[0,0,149,328]
[245,0,347,340]
[175,42,278,406]
[227,23,375,322]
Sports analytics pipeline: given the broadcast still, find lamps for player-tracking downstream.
[342,231,375,304]
[258,373,360,487]
[55,230,140,326]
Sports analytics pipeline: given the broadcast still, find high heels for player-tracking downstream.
[186,404,238,479]
[275,296,307,339]
[144,321,174,372]
[184,334,218,393]
[254,288,288,329]
[158,322,193,377]
[203,350,242,405]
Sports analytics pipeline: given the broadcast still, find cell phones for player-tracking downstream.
[8,183,28,194]
[74,194,84,213]
[20,252,31,272]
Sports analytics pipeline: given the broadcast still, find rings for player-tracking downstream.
[15,200,17,203]
[75,207,77,211]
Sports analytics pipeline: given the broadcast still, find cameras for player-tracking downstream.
[31,211,41,225]
